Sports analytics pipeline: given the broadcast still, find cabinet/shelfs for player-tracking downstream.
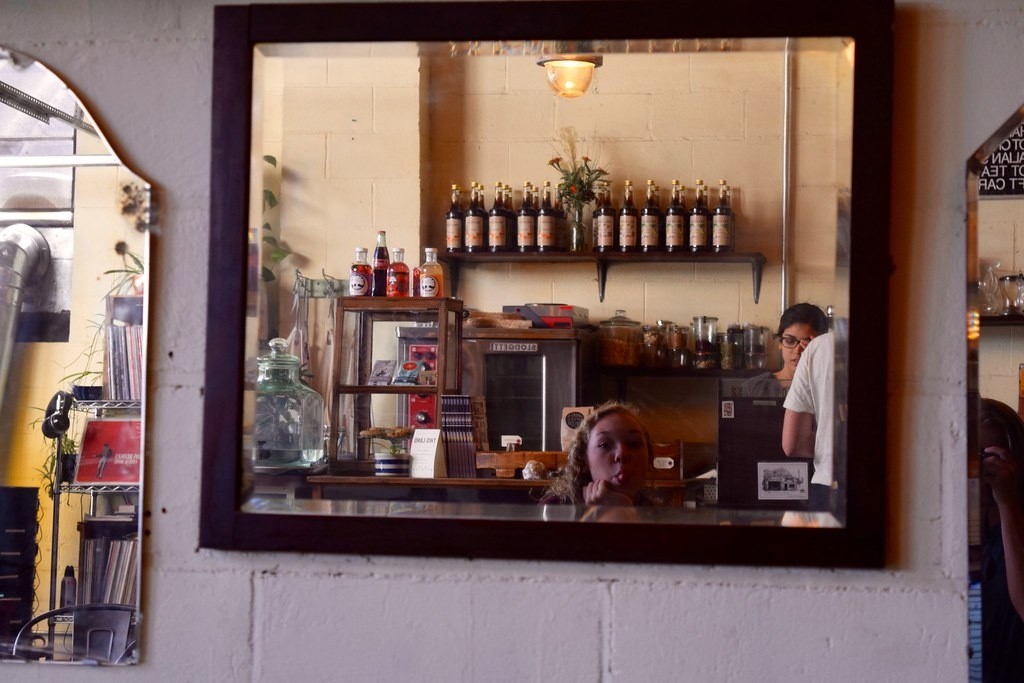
[325,291,462,464]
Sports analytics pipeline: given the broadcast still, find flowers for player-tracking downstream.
[547,125,611,246]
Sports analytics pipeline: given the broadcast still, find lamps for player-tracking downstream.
[536,52,603,99]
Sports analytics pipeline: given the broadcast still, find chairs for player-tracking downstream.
[13,603,137,662]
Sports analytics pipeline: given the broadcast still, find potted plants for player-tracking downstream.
[98,248,143,297]
[27,417,79,497]
[371,440,412,476]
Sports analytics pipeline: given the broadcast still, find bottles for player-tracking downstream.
[592,178,734,253]
[349,247,372,296]
[372,230,391,295]
[599,310,769,370]
[59,564,76,616]
[254,338,326,468]
[978,262,1024,315]
[419,248,443,298]
[386,248,409,298]
[446,181,567,252]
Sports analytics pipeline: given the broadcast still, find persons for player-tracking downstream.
[93,444,112,478]
[979,398,1024,683]
[782,331,835,509]
[537,399,656,522]
[737,303,828,399]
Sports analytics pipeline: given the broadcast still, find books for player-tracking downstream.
[393,360,423,384]
[369,359,397,384]
[70,323,142,606]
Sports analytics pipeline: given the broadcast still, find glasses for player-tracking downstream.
[780,335,815,348]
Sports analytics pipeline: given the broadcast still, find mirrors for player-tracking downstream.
[199,1,896,571]
[965,102,1023,683]
[0,48,157,663]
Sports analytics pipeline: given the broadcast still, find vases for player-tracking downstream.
[569,206,586,252]
